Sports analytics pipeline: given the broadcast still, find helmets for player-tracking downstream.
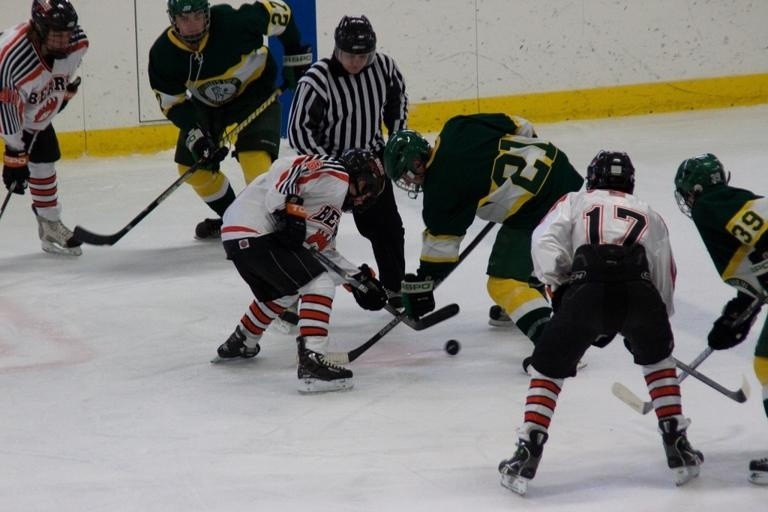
[339,146,385,215]
[384,131,432,199]
[31,1,80,57]
[588,151,636,195]
[168,0,212,44]
[675,153,728,219]
[334,15,377,55]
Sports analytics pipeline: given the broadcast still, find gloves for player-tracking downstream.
[708,290,760,350]
[400,273,436,321]
[59,84,77,113]
[349,271,387,312]
[2,146,32,195]
[295,48,310,78]
[183,124,222,171]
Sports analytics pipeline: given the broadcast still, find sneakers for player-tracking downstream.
[489,305,509,322]
[750,457,768,470]
[38,213,75,246]
[280,302,301,325]
[297,349,353,381]
[661,432,705,467]
[500,440,544,480]
[196,217,223,238]
[218,335,261,356]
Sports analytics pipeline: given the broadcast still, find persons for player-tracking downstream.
[385,112,588,377]
[0,0,89,257]
[673,154,768,486]
[209,149,388,393]
[287,15,408,308]
[499,151,705,496]
[148,0,313,238]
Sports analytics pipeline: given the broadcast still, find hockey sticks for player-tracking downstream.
[674,356,751,403]
[327,220,497,366]
[612,346,715,415]
[74,82,290,246]
[302,240,459,331]
